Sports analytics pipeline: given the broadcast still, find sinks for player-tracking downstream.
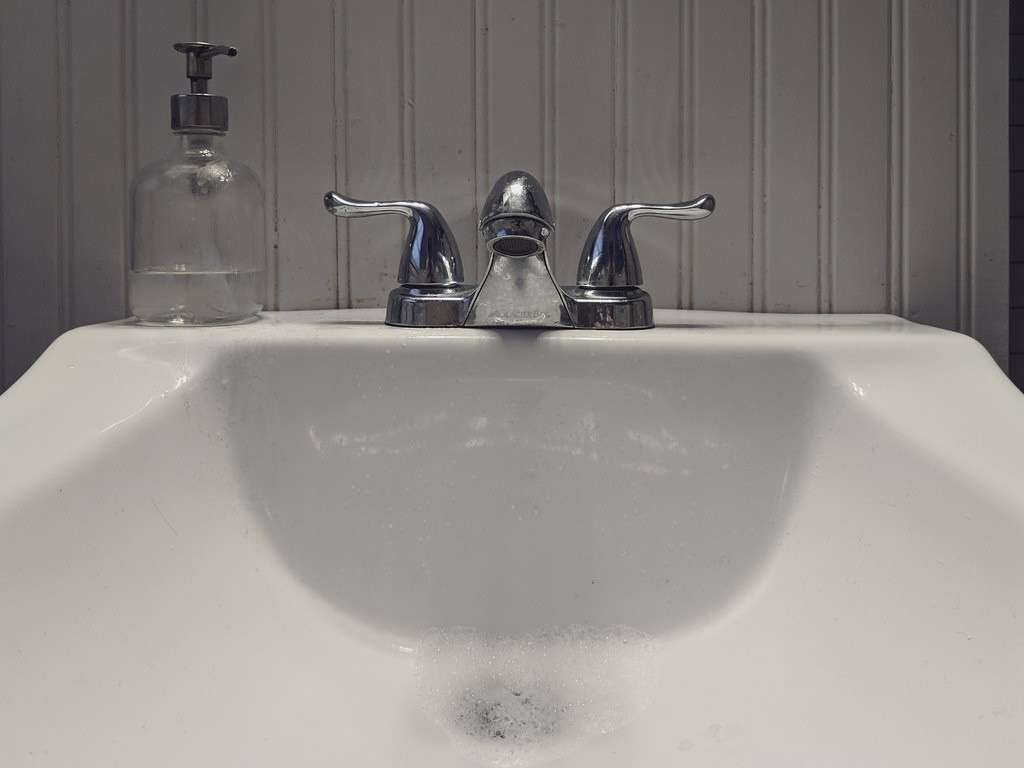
[0,301,1024,768]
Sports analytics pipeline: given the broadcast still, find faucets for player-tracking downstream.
[466,167,575,330]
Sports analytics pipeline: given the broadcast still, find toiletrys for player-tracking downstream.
[122,36,275,327]
